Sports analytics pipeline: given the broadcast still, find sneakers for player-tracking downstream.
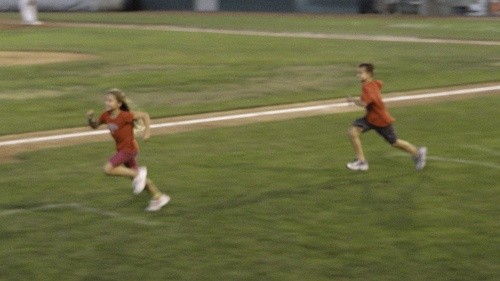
[412,146,426,170]
[345,158,369,171]
[144,193,171,211]
[132,166,147,194]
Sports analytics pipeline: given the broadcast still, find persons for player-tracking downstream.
[86,88,171,212]
[346,64,427,170]
[18,0,45,25]
